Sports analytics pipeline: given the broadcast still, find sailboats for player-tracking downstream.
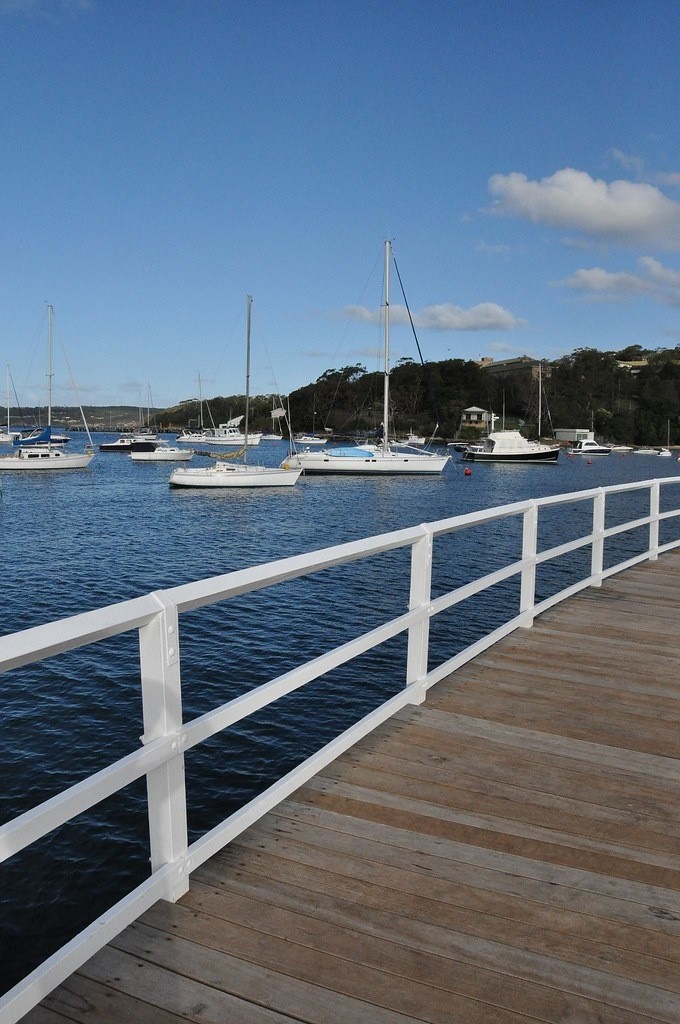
[0,363,328,452]
[0,303,97,469]
[277,240,454,475]
[167,294,304,487]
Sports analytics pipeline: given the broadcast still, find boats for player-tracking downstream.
[612,418,672,457]
[447,362,561,465]
[566,439,613,456]
[129,446,197,461]
[378,425,425,449]
